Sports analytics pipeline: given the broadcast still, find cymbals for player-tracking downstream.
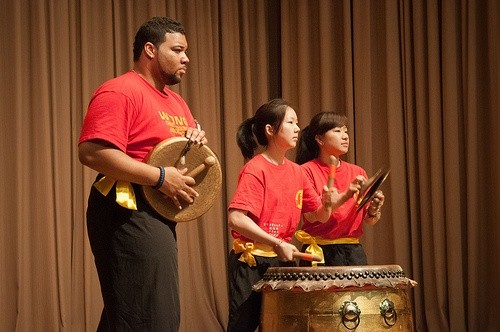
[353,165,392,215]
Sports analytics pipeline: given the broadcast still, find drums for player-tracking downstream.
[248,262,418,332]
[141,136,223,224]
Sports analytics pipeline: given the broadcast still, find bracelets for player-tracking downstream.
[366,205,377,219]
[151,166,165,190]
[273,238,285,251]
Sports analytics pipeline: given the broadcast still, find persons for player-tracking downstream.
[294,112,385,267]
[227,98,338,332]
[77,15,208,332]
